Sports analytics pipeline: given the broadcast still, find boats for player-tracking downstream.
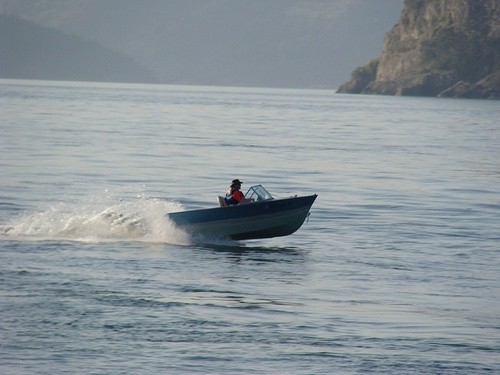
[166,183,319,241]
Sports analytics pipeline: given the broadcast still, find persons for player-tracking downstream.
[230,179,254,203]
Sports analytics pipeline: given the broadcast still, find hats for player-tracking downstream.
[230,179,243,187]
